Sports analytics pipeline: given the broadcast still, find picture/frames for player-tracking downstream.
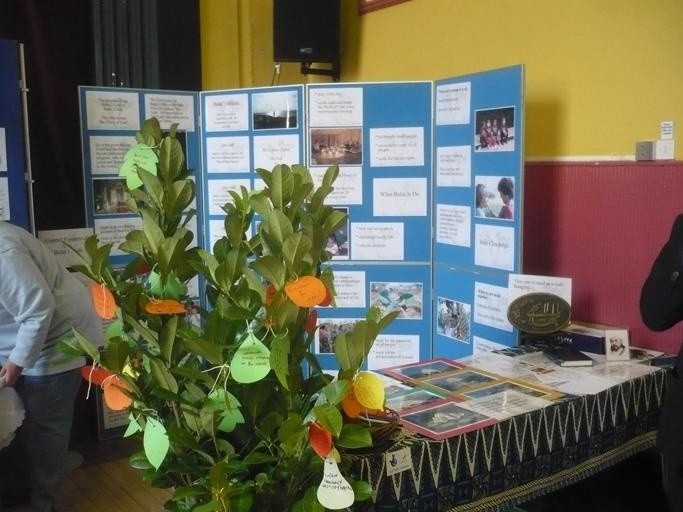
[356,0,412,17]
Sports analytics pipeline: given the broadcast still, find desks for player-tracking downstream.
[321,325,683,512]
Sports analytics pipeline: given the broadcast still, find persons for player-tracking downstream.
[639,213,683,512]
[609,337,626,356]
[480,117,509,150]
[476,184,499,218]
[497,178,514,220]
[112,181,129,214]
[0,221,92,511]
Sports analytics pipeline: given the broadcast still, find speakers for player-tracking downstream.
[273,0,340,63]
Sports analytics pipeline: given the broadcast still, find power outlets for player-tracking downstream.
[633,140,656,164]
[655,137,676,161]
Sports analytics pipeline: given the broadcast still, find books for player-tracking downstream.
[542,347,593,367]
